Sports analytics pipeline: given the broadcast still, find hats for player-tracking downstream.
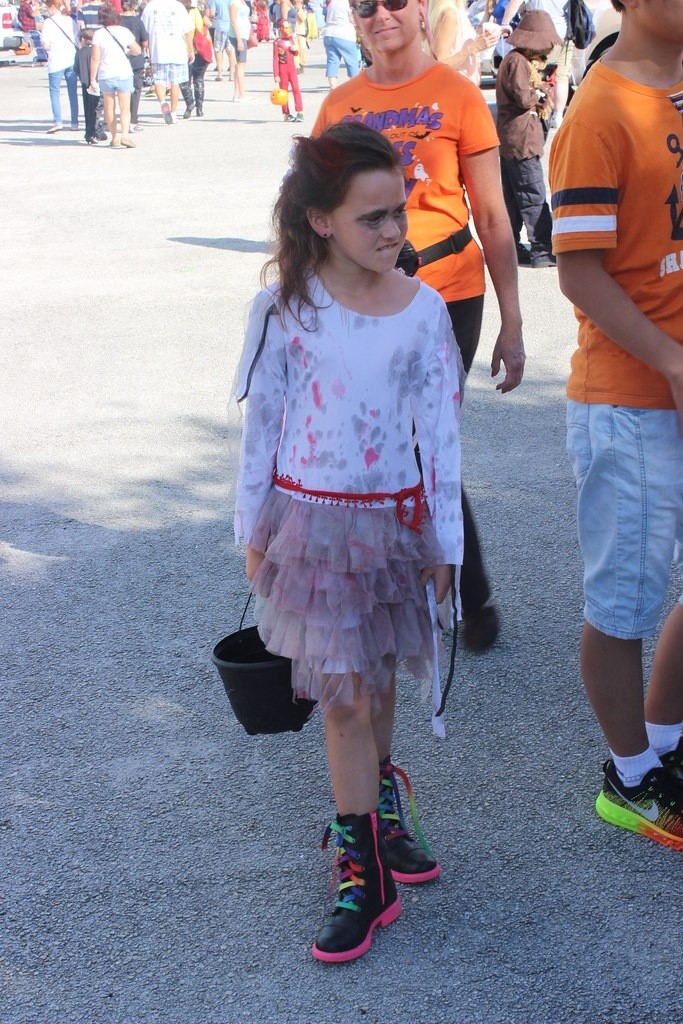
[506,10,564,50]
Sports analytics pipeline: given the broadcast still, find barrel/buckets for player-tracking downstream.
[209,589,315,736]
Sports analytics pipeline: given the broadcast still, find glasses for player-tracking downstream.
[352,0,407,17]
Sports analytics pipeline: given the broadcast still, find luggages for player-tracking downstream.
[257,16,269,42]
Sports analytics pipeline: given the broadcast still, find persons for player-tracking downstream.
[309,0,524,650]
[495,10,556,267]
[546,0,683,851]
[231,119,467,964]
[15,0,606,155]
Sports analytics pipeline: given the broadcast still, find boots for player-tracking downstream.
[195,83,204,116]
[312,814,402,962]
[376,755,439,883]
[180,83,194,118]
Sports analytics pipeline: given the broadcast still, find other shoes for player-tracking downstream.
[166,89,171,95]
[213,66,234,81]
[534,254,555,267]
[460,581,499,655]
[145,90,156,96]
[46,102,178,149]
[515,243,531,264]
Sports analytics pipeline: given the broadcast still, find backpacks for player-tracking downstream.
[563,0,595,49]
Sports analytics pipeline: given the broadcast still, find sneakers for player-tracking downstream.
[285,115,295,122]
[296,113,303,122]
[596,759,683,850]
[658,736,683,779]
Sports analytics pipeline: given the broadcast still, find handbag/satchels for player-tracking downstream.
[193,32,212,62]
[395,240,418,278]
[2,12,32,56]
[249,27,257,49]
[74,52,80,75]
